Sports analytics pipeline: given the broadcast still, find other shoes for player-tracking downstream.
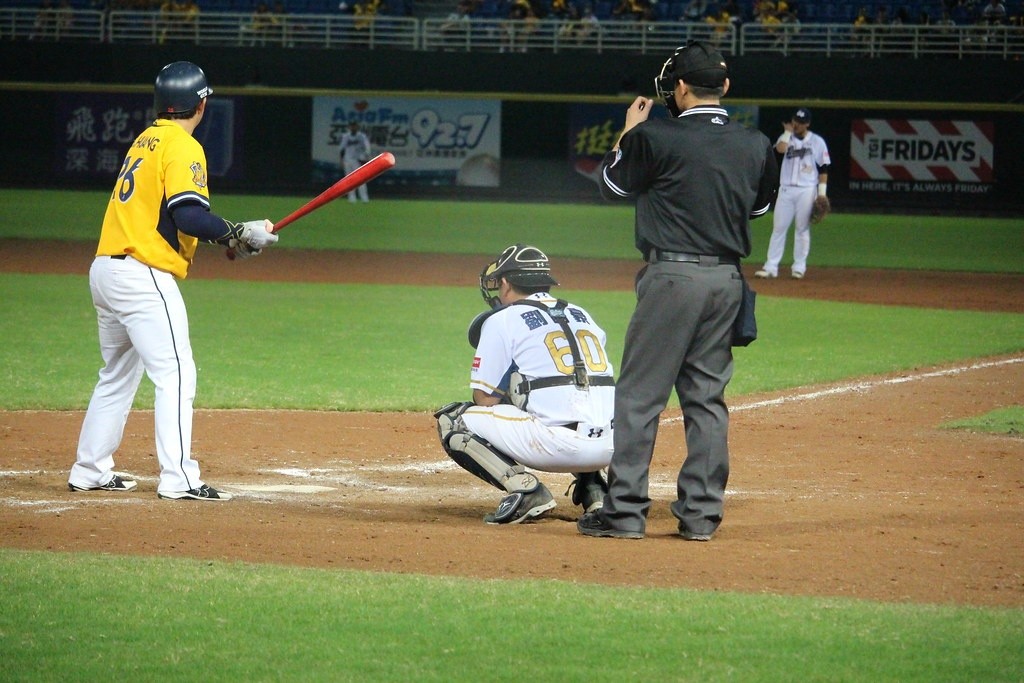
[755,269,777,279]
[791,272,801,278]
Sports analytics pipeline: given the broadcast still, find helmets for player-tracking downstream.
[486,244,561,287]
[155,61,214,114]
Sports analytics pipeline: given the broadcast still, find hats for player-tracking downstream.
[669,40,729,79]
[349,120,356,125]
[792,108,811,123]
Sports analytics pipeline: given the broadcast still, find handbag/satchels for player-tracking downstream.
[731,266,757,346]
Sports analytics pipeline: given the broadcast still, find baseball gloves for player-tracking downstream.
[809,196,830,225]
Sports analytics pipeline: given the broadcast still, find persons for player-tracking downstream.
[551,0,603,52]
[917,12,931,46]
[433,244,618,524]
[612,0,665,51]
[755,0,802,56]
[30,3,74,38]
[156,0,200,45]
[69,61,279,504]
[681,0,735,52]
[497,0,539,53]
[936,11,957,57]
[964,0,1024,59]
[439,0,484,51]
[338,120,371,201]
[577,43,777,541]
[850,6,910,56]
[251,0,308,47]
[344,0,389,48]
[755,107,832,279]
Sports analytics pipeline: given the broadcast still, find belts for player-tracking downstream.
[563,419,615,438]
[643,251,737,265]
[111,255,126,260]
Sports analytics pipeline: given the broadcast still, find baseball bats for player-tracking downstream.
[227,151,395,261]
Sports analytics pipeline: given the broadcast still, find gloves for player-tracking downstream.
[239,220,277,251]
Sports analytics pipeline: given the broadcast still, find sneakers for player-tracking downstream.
[576,512,645,539]
[678,528,711,541]
[483,482,557,525]
[68,474,138,492]
[158,484,232,502]
[565,473,608,513]
